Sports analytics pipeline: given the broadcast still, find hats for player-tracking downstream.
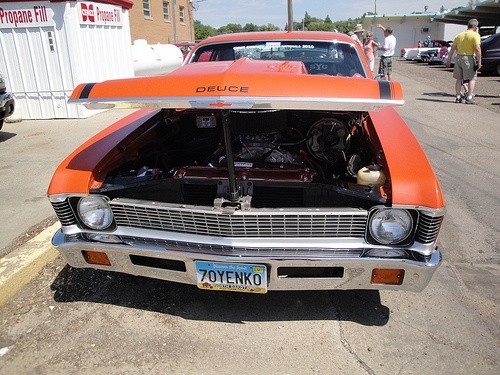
[353,25,365,33]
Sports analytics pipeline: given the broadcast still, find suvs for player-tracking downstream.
[476,32,500,76]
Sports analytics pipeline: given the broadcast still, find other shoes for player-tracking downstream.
[465,96,478,104]
[456,94,462,102]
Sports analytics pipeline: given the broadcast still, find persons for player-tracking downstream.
[362,31,380,75]
[418,40,423,48]
[446,18,482,104]
[350,23,365,57]
[376,24,396,83]
[424,34,431,47]
[330,28,354,60]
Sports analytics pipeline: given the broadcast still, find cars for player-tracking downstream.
[173,41,213,62]
[400,39,456,68]
[46,27,446,294]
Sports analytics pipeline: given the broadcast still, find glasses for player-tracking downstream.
[366,36,371,38]
